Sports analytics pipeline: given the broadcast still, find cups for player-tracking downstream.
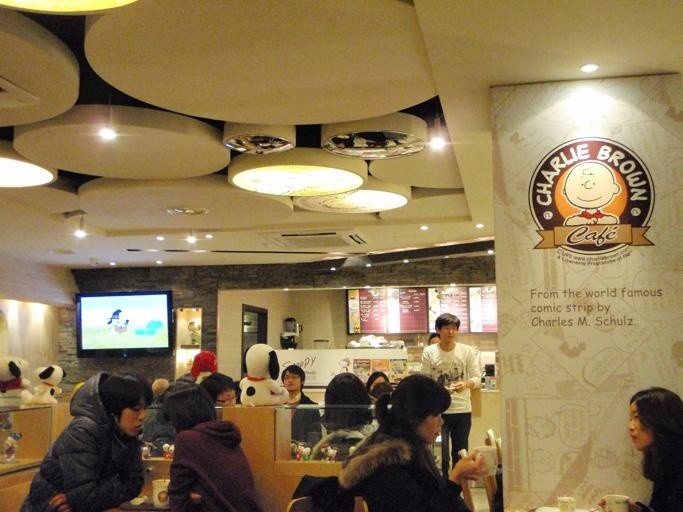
[604,494,628,512]
[151,479,172,507]
[558,497,576,511]
[476,445,499,476]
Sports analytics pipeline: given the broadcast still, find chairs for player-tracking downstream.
[285,493,365,512]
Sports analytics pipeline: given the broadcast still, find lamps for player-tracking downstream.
[292,175,412,215]
[225,145,370,198]
[0,139,58,188]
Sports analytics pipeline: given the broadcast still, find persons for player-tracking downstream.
[484,434,503,512]
[304,475,357,512]
[428,334,444,345]
[594,384,683,511]
[17,370,153,512]
[288,373,372,500]
[419,313,481,485]
[231,379,243,400]
[337,375,487,512]
[150,351,393,446]
[156,385,261,511]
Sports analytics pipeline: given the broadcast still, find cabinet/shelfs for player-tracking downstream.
[0,401,75,512]
[140,403,382,512]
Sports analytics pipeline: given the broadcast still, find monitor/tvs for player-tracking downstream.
[76,290,173,358]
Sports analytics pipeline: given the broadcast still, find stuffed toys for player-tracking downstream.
[0,355,32,398]
[188,321,201,344]
[3,432,22,460]
[0,414,12,428]
[19,365,67,405]
[279,317,303,350]
[239,342,290,405]
